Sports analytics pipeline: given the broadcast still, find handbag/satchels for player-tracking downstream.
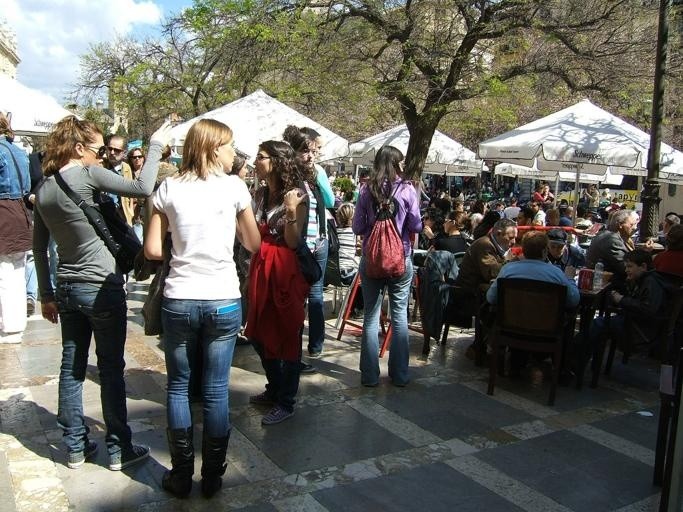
[52,169,143,274]
[326,218,340,254]
[277,235,322,286]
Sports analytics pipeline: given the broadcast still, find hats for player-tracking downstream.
[546,228,572,245]
[666,212,681,225]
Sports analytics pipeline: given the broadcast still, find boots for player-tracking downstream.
[199,428,232,498]
[162,420,195,495]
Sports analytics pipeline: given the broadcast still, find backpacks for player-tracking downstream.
[364,178,406,280]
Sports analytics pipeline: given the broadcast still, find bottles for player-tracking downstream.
[592,259,604,289]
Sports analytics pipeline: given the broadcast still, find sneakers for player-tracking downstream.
[0,298,35,344]
[249,390,277,405]
[261,404,295,425]
[107,445,151,471]
[66,439,99,468]
[299,360,316,372]
[308,344,322,357]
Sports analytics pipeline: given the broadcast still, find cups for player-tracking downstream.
[511,247,522,256]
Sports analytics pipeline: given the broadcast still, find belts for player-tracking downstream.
[56,276,123,287]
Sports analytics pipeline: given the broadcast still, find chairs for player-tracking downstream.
[307,214,682,408]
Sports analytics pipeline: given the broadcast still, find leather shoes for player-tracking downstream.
[465,344,483,372]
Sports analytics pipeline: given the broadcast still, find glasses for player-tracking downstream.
[87,145,107,156]
[132,156,144,159]
[256,156,271,161]
[108,147,124,153]
[422,216,430,220]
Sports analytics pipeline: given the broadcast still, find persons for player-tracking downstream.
[1,118,331,358]
[144,117,261,498]
[249,140,308,425]
[332,168,682,388]
[33,117,151,470]
